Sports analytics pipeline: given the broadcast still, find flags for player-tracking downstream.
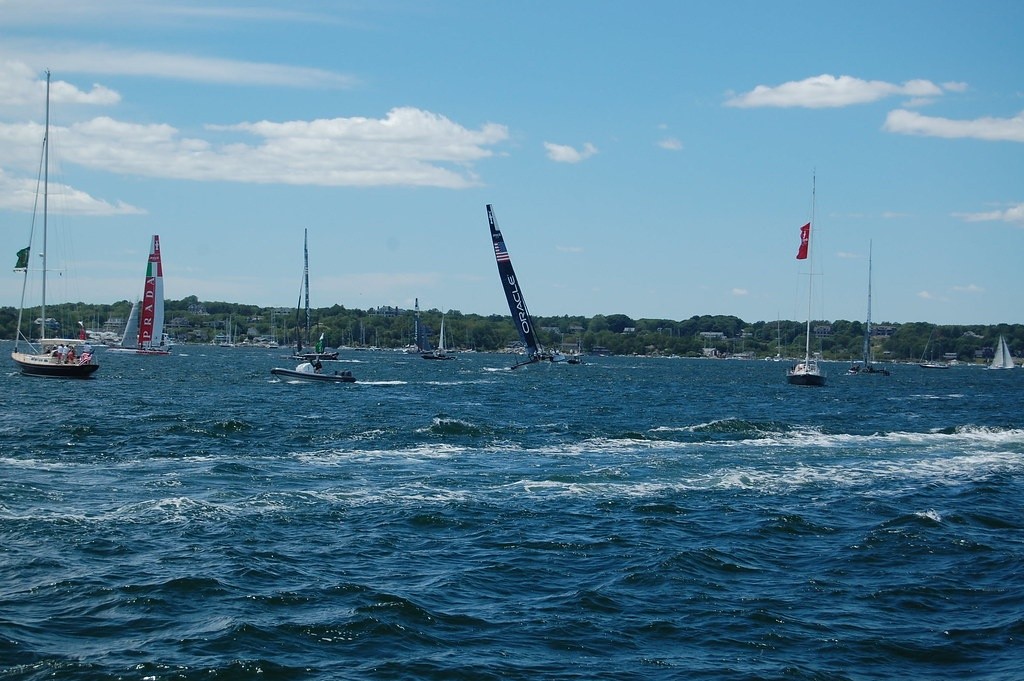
[13,246,30,272]
[796,222,809,259]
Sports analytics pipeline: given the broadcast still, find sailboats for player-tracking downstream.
[982,332,1015,371]
[918,322,949,368]
[784,167,828,385]
[486,204,564,372]
[104,233,171,355]
[280,227,339,361]
[219,314,233,347]
[843,240,888,376]
[403,297,433,354]
[267,309,279,349]
[10,70,100,379]
[421,308,457,359]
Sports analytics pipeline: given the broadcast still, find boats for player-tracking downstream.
[37,336,101,346]
[566,358,583,364]
[270,366,357,384]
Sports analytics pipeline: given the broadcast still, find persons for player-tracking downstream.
[83,342,92,363]
[57,343,63,362]
[62,344,69,362]
[313,358,322,373]
